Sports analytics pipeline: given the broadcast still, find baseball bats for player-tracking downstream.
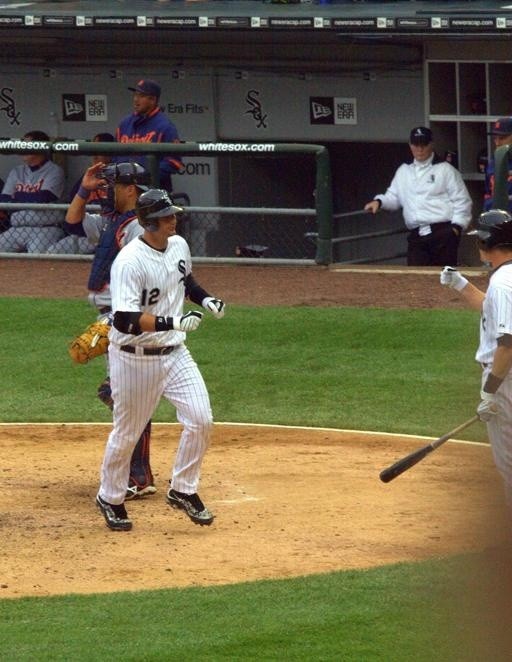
[380,405,498,483]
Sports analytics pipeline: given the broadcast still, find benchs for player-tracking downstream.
[0,154,219,257]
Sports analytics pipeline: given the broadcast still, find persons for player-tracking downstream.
[438,209,512,505]
[482,117,512,212]
[0,130,66,253]
[110,78,182,195]
[96,188,226,533]
[62,161,157,504]
[44,133,115,254]
[364,127,474,267]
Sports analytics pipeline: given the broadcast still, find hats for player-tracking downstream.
[488,117,512,137]
[126,79,162,97]
[410,126,432,146]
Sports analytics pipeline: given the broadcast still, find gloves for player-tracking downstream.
[172,310,204,333]
[440,265,468,294]
[203,296,226,321]
[478,390,499,422]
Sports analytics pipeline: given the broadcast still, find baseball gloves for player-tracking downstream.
[69,318,112,364]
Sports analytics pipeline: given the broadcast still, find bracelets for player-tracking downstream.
[76,184,90,201]
[155,316,173,332]
[482,373,504,394]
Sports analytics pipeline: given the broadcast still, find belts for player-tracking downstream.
[119,344,179,356]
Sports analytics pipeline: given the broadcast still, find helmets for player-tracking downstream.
[95,163,147,185]
[136,188,182,232]
[466,207,512,249]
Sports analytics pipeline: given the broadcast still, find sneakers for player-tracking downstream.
[95,491,133,531]
[124,481,158,502]
[167,489,214,525]
